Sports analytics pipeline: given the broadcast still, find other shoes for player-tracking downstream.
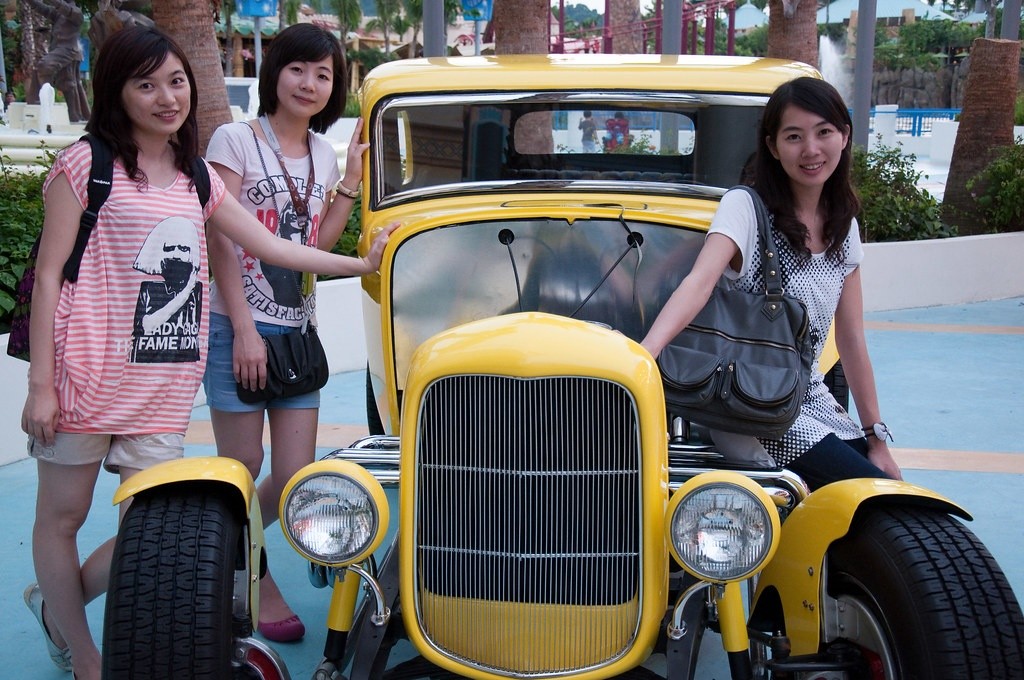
[23,583,73,672]
[258,615,305,642]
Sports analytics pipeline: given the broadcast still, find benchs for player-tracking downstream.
[502,168,707,185]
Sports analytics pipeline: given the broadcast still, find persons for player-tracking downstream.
[207,24,373,645]
[25,24,399,680]
[639,77,903,489]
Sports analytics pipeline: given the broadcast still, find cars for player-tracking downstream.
[101,56,1024,680]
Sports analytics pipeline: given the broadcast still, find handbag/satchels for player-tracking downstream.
[654,185,813,440]
[237,321,329,403]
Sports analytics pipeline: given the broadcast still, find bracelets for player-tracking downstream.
[861,422,894,443]
[334,180,362,200]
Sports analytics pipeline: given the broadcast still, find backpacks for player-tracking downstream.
[7,132,212,362]
